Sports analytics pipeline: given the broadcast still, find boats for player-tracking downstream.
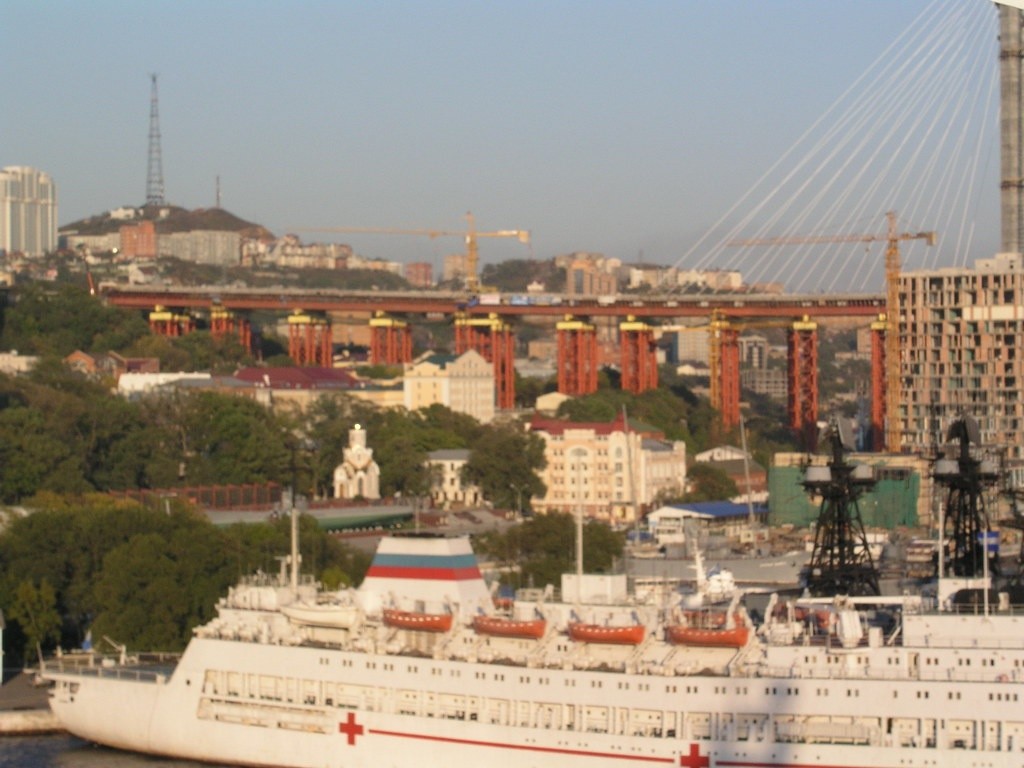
[666,626,749,647]
[383,609,454,632]
[570,620,645,647]
[474,616,545,639]
[280,605,357,628]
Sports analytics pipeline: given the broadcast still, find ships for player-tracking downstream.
[23,508,1023,768]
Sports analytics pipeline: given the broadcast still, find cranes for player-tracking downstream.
[726,212,939,452]
[286,211,530,290]
